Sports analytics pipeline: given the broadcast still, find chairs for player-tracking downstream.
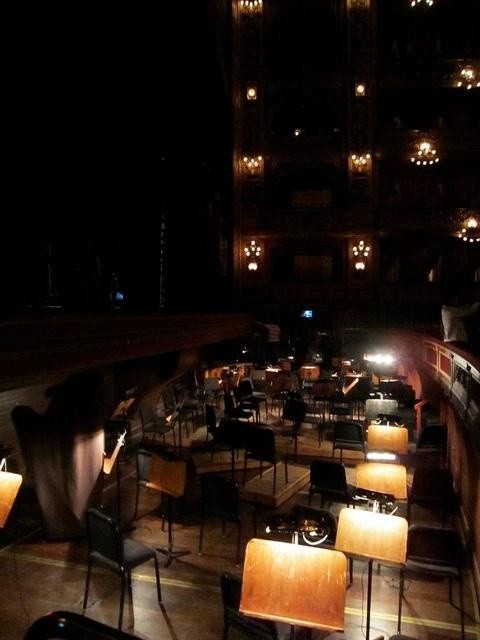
[22,362,465,640]
